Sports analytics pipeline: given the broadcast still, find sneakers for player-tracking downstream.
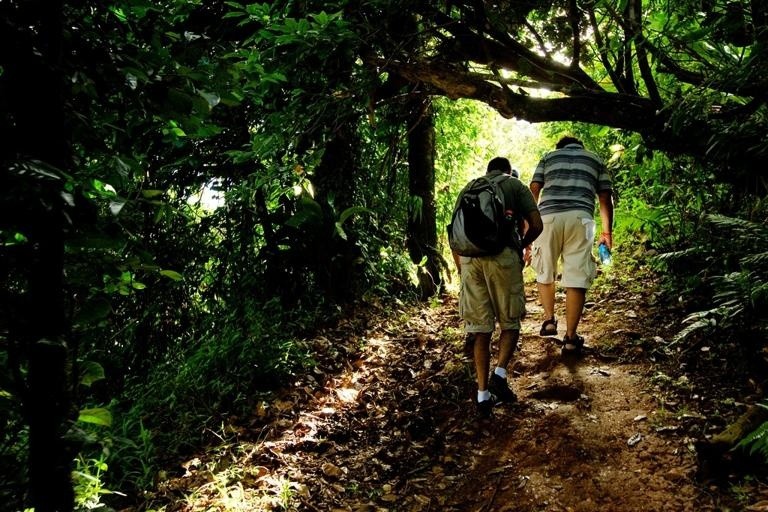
[476,392,499,417]
[487,371,516,403]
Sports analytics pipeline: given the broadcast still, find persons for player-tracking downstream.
[447,155,544,416]
[528,136,615,359]
[459,166,533,366]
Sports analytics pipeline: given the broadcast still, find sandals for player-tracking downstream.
[560,334,585,354]
[540,318,558,337]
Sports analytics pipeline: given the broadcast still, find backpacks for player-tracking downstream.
[446,172,512,258]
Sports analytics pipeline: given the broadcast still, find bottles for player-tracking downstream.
[599,243,611,266]
